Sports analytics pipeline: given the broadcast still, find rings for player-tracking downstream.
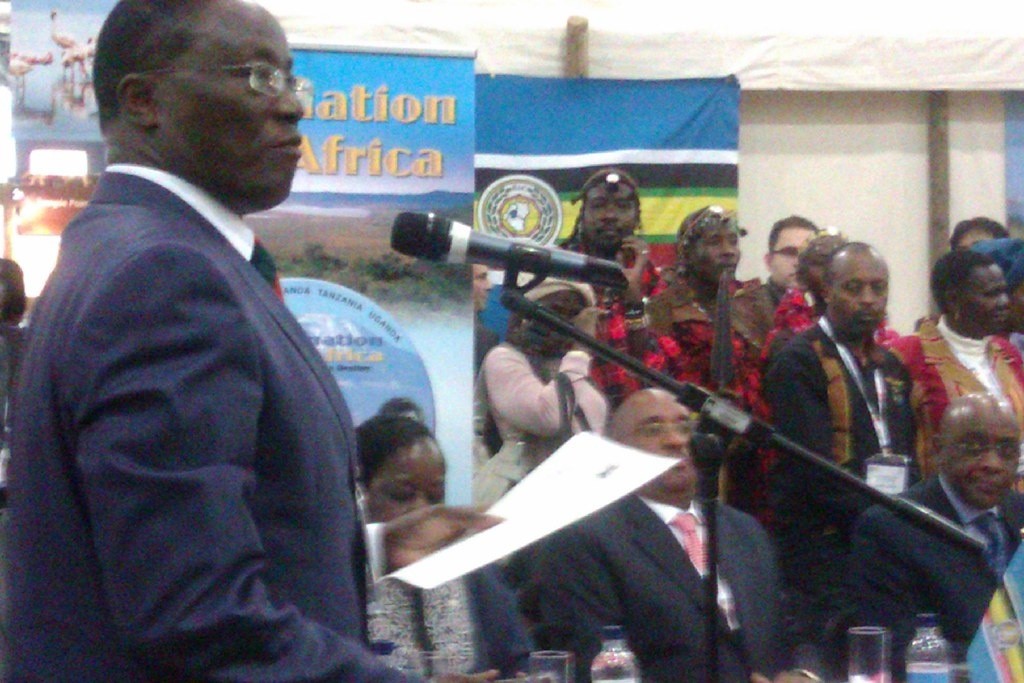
[643,250,650,254]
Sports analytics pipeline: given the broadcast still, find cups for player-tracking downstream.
[530,651,575,683]
[848,627,892,683]
[418,652,464,679]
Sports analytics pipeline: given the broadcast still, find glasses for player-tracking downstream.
[771,245,798,257]
[138,60,312,108]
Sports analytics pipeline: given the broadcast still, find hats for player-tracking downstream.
[527,275,592,308]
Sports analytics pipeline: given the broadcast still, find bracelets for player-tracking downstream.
[623,301,644,332]
[797,669,819,680]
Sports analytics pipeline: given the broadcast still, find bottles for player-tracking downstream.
[905,614,954,683]
[368,640,400,672]
[592,626,641,683]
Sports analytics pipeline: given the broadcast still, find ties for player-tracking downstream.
[248,241,281,301]
[672,512,709,583]
[850,343,880,414]
[974,512,1011,585]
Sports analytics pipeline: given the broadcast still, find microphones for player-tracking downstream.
[390,212,628,290]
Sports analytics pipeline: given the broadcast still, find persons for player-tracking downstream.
[849,390,1024,677]
[480,273,610,483]
[647,203,1024,593]
[470,257,499,432]
[353,414,535,683]
[524,387,826,683]
[555,168,666,408]
[10,0,499,683]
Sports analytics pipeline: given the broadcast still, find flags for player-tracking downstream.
[473,72,741,337]
